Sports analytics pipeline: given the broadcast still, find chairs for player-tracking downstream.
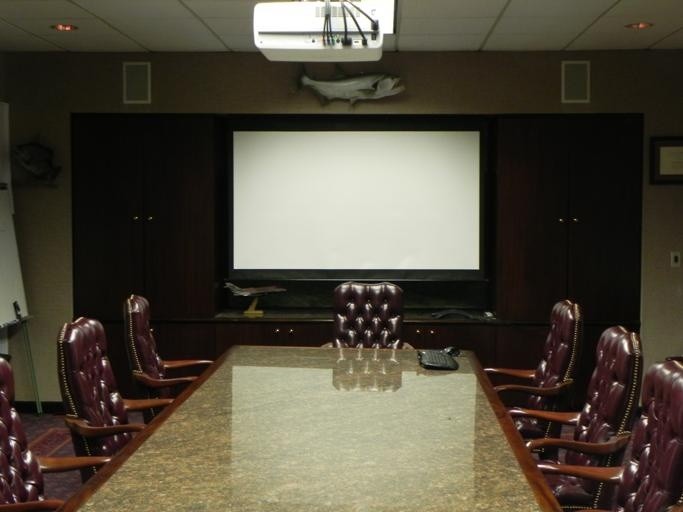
[529,354,682,510]
[54,314,177,479]
[318,278,417,350]
[506,324,646,466]
[0,356,115,512]
[492,303,583,417]
[123,293,212,423]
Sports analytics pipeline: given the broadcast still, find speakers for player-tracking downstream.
[122,61,151,104]
[560,61,591,104]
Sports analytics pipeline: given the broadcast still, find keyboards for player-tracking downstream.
[417,349,458,370]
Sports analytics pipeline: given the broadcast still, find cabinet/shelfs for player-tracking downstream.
[105,310,641,404]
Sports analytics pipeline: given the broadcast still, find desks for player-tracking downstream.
[53,341,571,512]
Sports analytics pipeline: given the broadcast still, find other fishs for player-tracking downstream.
[294,63,406,107]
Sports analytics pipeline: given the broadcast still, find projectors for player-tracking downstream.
[253,1,385,63]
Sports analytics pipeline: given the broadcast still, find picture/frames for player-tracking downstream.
[650,136,682,185]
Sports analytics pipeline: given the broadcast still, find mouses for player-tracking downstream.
[443,346,460,356]
